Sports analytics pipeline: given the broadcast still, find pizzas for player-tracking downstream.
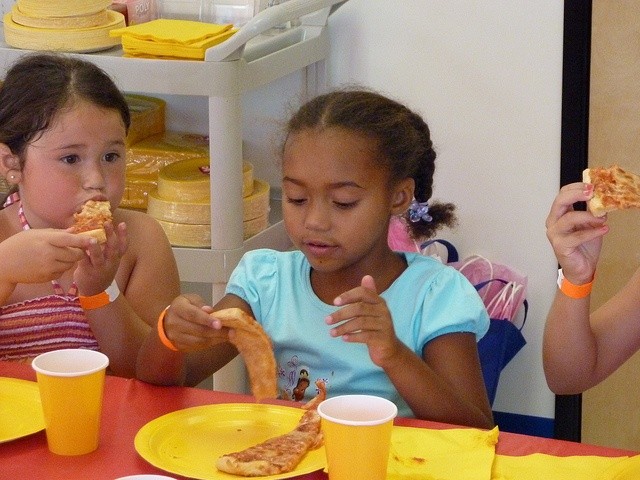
[217,380,326,475]
[74,200,112,244]
[208,308,277,400]
[583,166,640,217]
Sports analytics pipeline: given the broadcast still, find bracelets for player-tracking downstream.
[78,280,123,310]
[556,268,595,298]
[158,304,180,352]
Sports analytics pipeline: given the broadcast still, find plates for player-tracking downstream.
[133,402,325,479]
[2,371,47,443]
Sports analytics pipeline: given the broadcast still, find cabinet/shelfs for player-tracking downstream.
[0,0,349,393]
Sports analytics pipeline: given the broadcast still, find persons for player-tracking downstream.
[543,182,639,395]
[1,56,181,378]
[136,90,497,431]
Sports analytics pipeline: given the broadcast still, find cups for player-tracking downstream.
[319,395,397,480]
[29,348,109,456]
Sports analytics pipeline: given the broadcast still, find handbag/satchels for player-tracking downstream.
[420,239,528,410]
